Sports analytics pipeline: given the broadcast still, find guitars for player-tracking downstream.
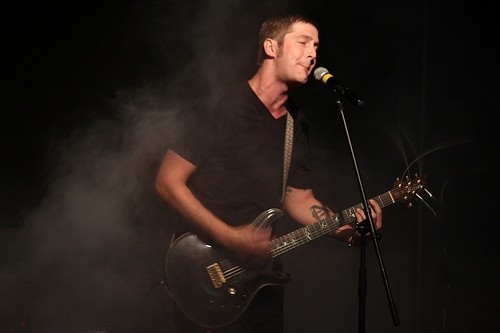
[163,168,433,329]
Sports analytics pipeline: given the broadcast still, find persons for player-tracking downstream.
[155,8,383,333]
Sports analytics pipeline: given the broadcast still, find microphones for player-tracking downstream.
[314,66,366,110]
